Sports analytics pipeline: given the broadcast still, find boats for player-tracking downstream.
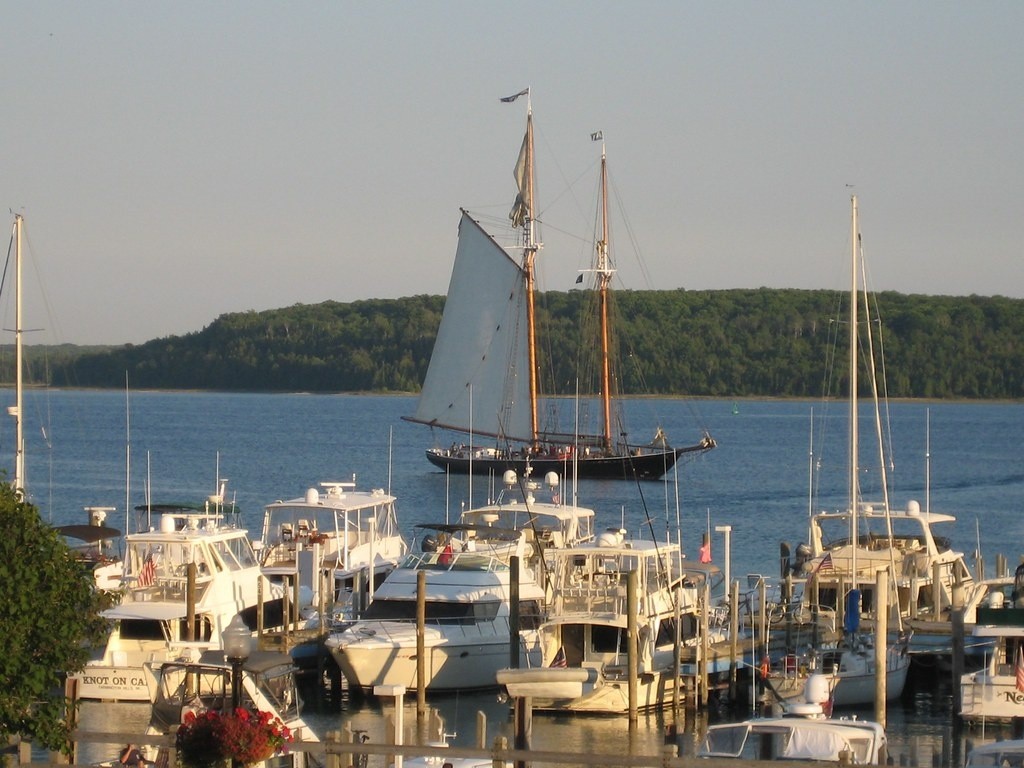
[1,188,1024,768]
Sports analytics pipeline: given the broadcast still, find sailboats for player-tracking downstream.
[400,86,719,481]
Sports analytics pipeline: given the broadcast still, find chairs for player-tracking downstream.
[282,522,293,542]
[521,529,535,543]
[298,519,310,535]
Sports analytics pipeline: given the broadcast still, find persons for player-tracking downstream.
[119,743,145,768]
[448,442,642,459]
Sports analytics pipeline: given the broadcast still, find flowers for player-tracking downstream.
[176,708,294,768]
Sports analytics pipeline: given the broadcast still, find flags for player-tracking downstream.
[700,544,712,563]
[1016,636,1024,693]
[548,648,567,669]
[805,552,833,584]
[437,542,452,565]
[135,550,156,587]
[499,87,529,102]
